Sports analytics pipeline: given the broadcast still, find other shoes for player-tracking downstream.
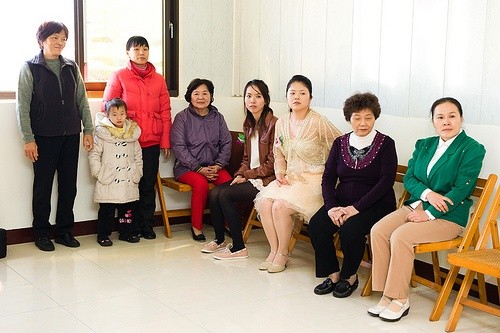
[201,239,226,252]
[224,227,231,236]
[211,244,248,259]
[379,298,410,322]
[191,226,206,242]
[119,232,140,242]
[140,229,156,239]
[367,294,392,316]
[97,235,113,246]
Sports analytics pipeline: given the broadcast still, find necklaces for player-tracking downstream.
[291,117,303,125]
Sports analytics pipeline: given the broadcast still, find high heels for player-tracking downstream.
[268,251,290,273]
[258,250,277,271]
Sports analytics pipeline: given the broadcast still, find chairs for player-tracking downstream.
[242,164,500,332]
[155,170,217,238]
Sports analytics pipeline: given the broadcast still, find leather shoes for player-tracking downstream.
[333,275,359,297]
[34,235,55,251]
[54,235,80,247]
[313,273,342,295]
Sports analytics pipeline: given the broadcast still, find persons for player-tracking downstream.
[200,79,280,259]
[16,20,94,251]
[307,92,398,297]
[366,96,487,322]
[252,74,343,273]
[101,35,172,240]
[88,96,143,246]
[171,77,233,242]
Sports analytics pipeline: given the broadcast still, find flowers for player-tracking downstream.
[274,137,284,148]
[235,133,246,145]
